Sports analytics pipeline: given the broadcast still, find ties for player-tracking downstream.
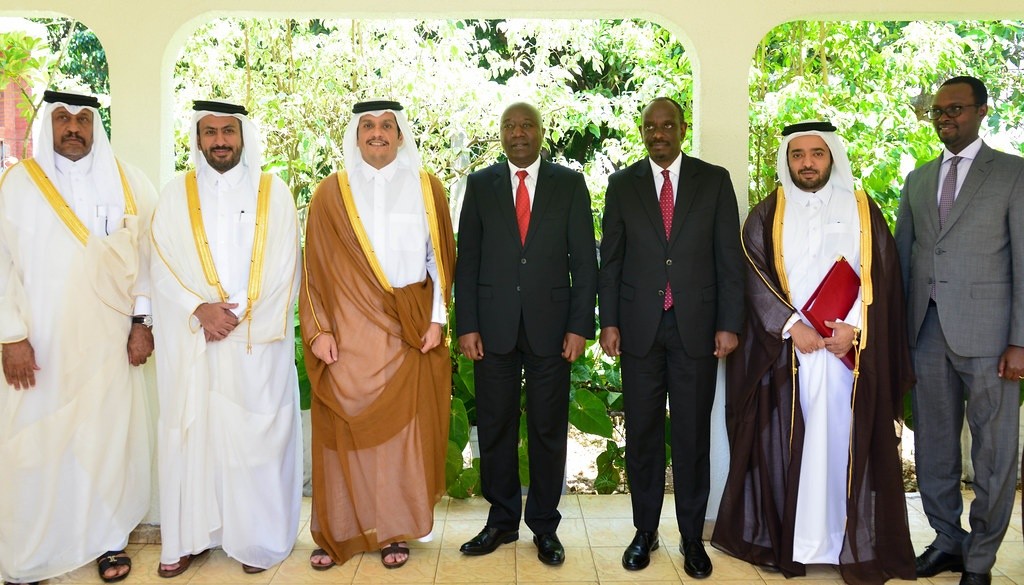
[515,170,530,248]
[659,169,674,307]
[931,156,964,301]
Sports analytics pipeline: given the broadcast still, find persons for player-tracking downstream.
[297,100,456,570]
[597,97,743,578]
[454,104,597,564]
[149,103,303,577]
[710,120,917,585]
[896,76,1024,585]
[0,91,156,584]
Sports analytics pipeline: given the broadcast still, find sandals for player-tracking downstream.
[4,580,40,585]
[97,548,131,583]
[381,541,409,569]
[242,563,265,574]
[158,553,195,578]
[311,547,336,570]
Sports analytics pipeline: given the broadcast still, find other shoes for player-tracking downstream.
[761,559,778,574]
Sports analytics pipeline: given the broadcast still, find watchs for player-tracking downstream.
[133,315,152,328]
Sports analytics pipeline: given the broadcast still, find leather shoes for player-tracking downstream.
[959,572,992,585]
[916,544,963,578]
[679,539,712,579]
[622,529,660,571]
[459,526,519,555]
[533,530,566,565]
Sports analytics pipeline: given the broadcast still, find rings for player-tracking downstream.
[1019,376,1024,379]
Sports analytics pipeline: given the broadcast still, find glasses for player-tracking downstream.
[925,104,983,120]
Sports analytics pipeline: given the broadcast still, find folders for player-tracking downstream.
[801,254,861,370]
